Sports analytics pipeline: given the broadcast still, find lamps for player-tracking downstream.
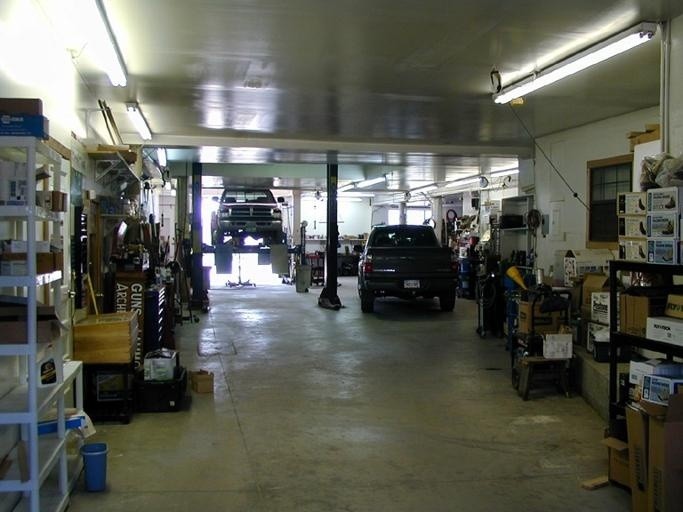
[487,19,660,106]
[85,0,130,92]
[124,101,154,142]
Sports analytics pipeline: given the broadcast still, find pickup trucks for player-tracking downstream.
[210,187,284,243]
[353,225,460,313]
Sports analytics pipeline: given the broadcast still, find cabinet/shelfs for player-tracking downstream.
[303,236,369,287]
[499,194,533,274]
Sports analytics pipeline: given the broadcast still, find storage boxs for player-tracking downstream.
[132,368,188,413]
[0,116,48,141]
[144,346,178,380]
[189,368,213,395]
[0,97,44,116]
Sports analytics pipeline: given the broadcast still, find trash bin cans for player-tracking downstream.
[202,267,212,289]
[296,265,311,292]
